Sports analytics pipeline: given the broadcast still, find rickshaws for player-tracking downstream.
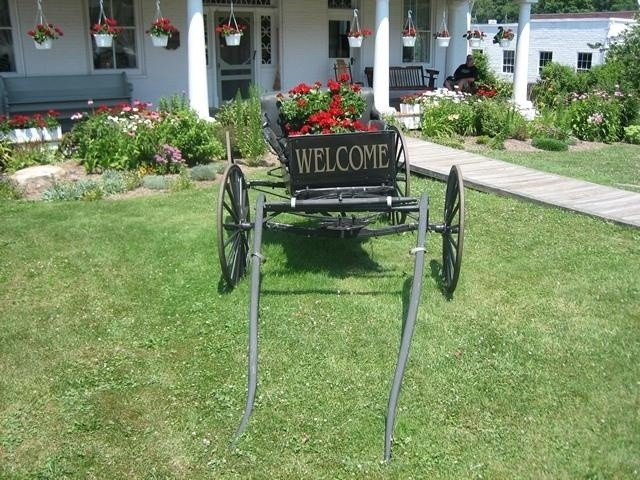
[202,90,465,467]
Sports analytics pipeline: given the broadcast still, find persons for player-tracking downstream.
[443,55,478,94]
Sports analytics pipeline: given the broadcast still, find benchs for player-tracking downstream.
[364,65,432,102]
[0,71,135,123]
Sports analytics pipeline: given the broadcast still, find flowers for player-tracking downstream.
[400,86,470,106]
[28,22,66,45]
[88,18,124,39]
[346,28,515,48]
[217,23,250,38]
[0,109,61,128]
[145,18,177,38]
[279,72,379,135]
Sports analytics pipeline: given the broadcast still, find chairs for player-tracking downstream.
[334,58,363,86]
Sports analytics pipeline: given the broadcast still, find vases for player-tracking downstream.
[151,33,167,47]
[96,34,114,48]
[226,33,242,47]
[0,125,64,153]
[35,40,51,50]
[399,104,425,130]
